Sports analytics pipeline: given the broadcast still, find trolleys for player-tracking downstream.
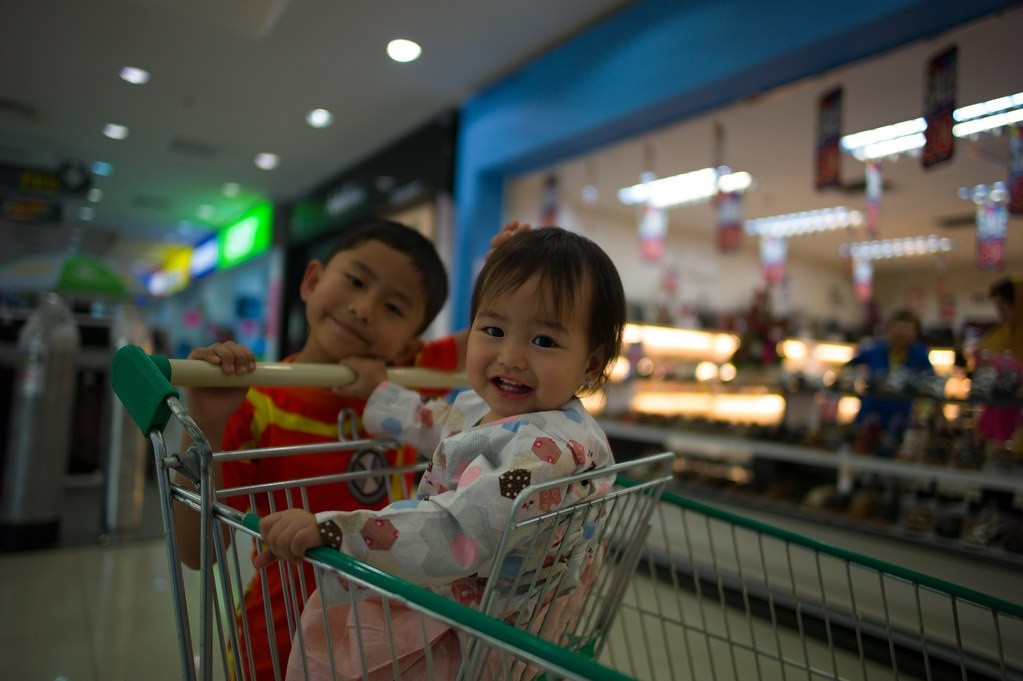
[106,341,1023,681]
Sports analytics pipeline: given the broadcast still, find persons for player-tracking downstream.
[174,222,535,681]
[252,226,624,681]
[832,274,1023,549]
